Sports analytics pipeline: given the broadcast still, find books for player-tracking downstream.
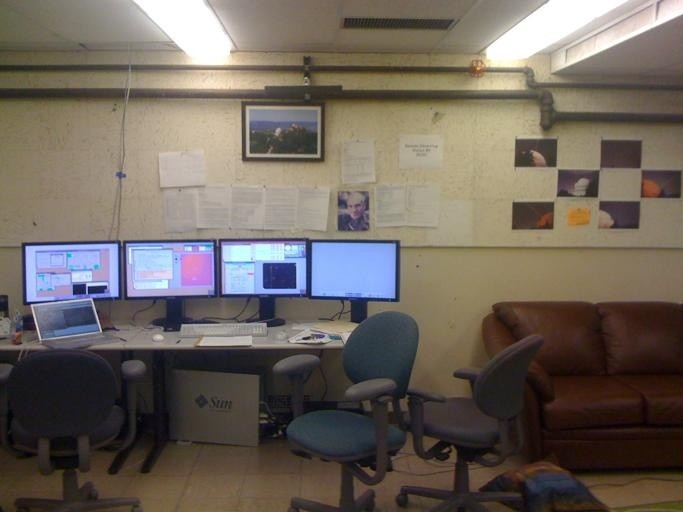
[288,319,360,345]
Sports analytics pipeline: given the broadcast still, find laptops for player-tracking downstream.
[30,297,120,349]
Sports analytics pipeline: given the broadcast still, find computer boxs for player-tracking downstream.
[164,365,264,447]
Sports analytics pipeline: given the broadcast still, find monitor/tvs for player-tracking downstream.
[219,237,308,326]
[22,241,122,305]
[123,238,218,326]
[308,238,400,323]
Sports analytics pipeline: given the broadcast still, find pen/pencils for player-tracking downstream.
[176,339,181,344]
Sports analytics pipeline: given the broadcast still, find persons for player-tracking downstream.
[337,192,370,231]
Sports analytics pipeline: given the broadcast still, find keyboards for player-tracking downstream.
[179,323,268,338]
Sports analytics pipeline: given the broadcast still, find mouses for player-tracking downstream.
[151,333,164,341]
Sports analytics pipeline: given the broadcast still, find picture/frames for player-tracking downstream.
[241,100,326,163]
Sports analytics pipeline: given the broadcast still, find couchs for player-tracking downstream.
[480,299,683,475]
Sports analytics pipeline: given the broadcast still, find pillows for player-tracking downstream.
[478,460,612,512]
